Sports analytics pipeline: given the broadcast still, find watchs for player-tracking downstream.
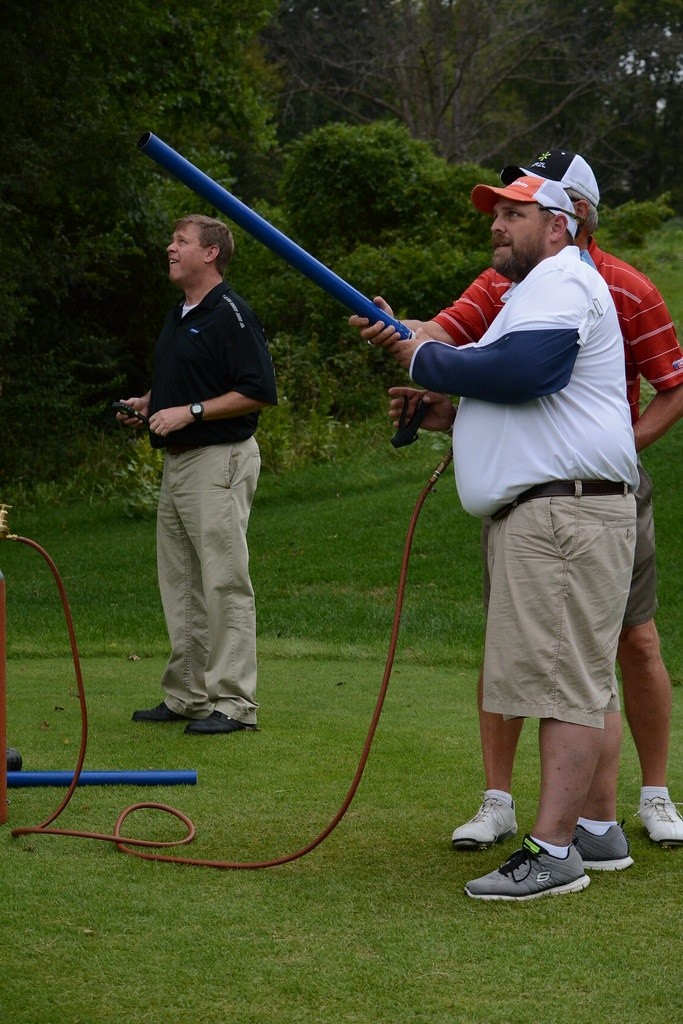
[190,402,204,421]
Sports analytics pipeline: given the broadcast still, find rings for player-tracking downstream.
[367,340,376,347]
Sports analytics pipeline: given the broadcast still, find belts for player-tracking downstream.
[490,480,632,521]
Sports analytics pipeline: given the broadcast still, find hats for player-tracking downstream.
[500,147,601,208]
[471,175,578,240]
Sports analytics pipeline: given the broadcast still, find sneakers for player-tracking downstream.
[462,833,591,901]
[579,824,634,871]
[452,791,519,848]
[184,710,257,735]
[634,796,683,847]
[131,701,190,722]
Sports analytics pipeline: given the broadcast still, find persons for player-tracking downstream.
[349,148,683,901]
[117,214,279,733]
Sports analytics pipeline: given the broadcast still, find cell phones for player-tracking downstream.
[113,402,149,422]
